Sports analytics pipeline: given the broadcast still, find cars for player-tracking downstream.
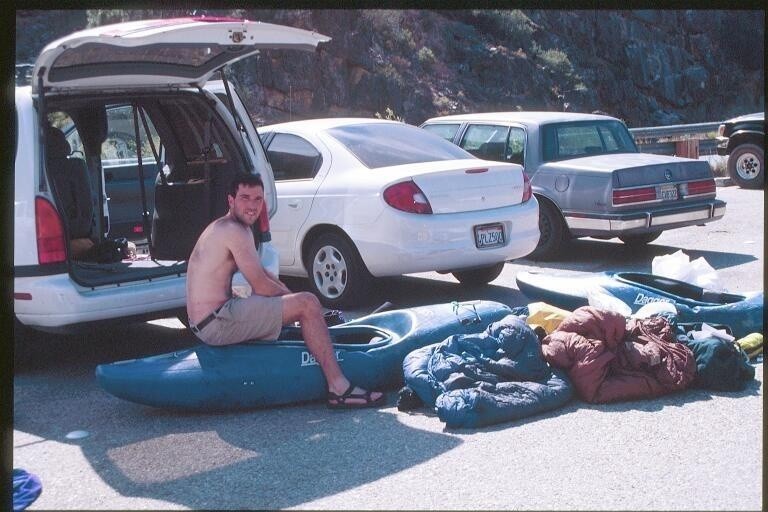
[418,111,728,261]
[214,116,542,310]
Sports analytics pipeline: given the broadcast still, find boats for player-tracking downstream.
[515,269,764,341]
[96,299,513,412]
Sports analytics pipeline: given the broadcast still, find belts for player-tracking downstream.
[192,306,223,333]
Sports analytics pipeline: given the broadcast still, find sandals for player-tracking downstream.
[327,384,384,406]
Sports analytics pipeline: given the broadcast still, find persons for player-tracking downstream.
[186,174,385,410]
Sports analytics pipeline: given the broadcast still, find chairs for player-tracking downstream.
[44,127,94,239]
[474,143,508,162]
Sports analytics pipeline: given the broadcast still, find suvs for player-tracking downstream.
[715,112,765,188]
[14,15,333,374]
